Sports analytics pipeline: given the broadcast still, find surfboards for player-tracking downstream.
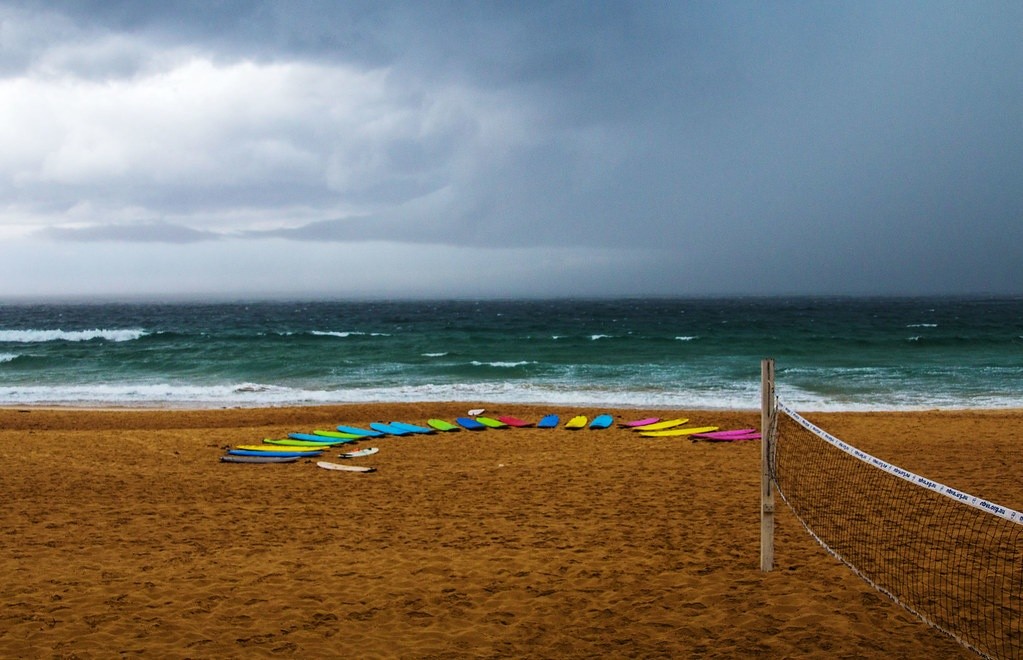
[216,403,763,475]
